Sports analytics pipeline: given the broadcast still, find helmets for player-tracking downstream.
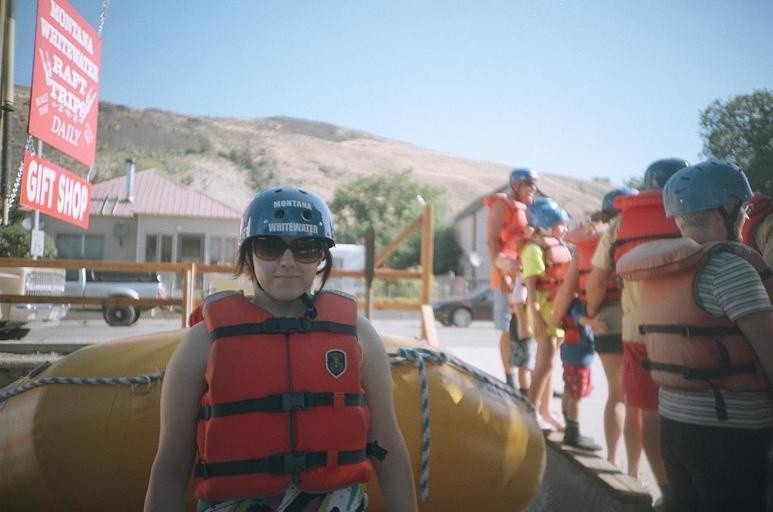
[511,160,755,227]
[237,186,334,250]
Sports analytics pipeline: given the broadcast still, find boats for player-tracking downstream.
[0,330,545,512]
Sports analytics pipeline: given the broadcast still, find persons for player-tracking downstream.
[138,186,422,512]
[488,158,773,511]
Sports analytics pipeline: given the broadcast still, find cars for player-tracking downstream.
[0,267,72,337]
[434,281,493,329]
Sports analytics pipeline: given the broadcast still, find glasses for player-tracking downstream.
[252,234,326,264]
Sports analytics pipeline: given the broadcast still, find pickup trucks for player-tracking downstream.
[63,268,163,327]
